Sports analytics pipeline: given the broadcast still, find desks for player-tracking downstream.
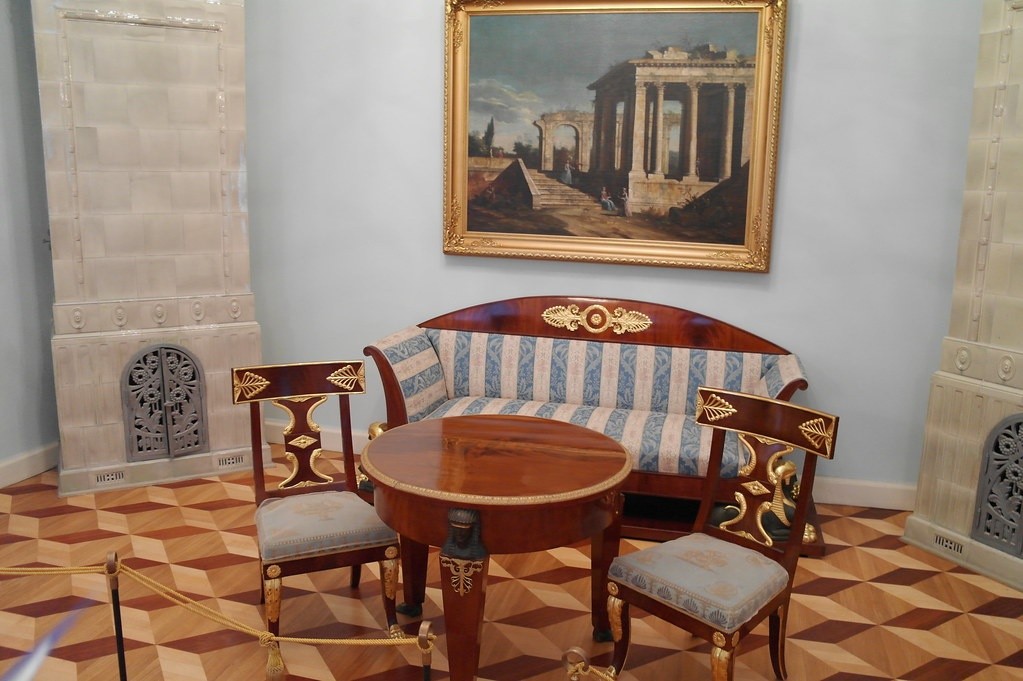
[360,414,633,681]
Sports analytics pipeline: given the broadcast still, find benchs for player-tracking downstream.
[364,296,826,560]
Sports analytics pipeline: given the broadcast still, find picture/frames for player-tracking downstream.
[443,0,787,274]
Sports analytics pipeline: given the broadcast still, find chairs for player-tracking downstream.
[231,360,405,681]
[607,386,838,681]
